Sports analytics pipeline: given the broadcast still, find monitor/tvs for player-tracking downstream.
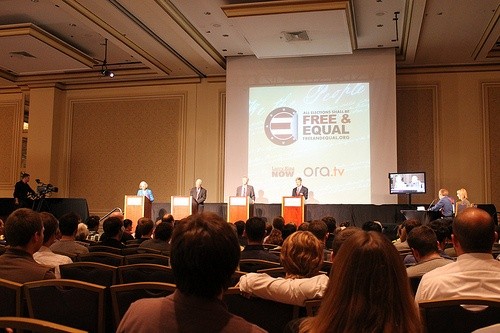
[389,172,426,194]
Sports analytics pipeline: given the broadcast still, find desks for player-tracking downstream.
[400,210,441,225]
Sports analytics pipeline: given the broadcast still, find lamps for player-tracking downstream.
[93,38,115,80]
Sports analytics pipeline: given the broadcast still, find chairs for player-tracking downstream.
[0,238,500,333]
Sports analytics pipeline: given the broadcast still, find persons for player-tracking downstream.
[392,174,407,190]
[136,181,155,203]
[455,188,470,205]
[234,176,256,204]
[137,222,172,257]
[235,226,363,306]
[408,176,422,189]
[74,211,176,249]
[308,220,332,262]
[292,178,308,201]
[413,207,500,310]
[404,221,457,266]
[117,211,268,333]
[238,216,281,263]
[263,217,309,251]
[0,208,56,300]
[283,230,425,333]
[13,173,35,207]
[405,225,455,277]
[361,218,454,252]
[322,216,338,250]
[279,231,324,280]
[428,188,454,222]
[50,211,89,258]
[227,220,247,251]
[190,179,207,214]
[32,211,73,280]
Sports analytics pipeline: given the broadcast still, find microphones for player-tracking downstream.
[427,199,436,210]
